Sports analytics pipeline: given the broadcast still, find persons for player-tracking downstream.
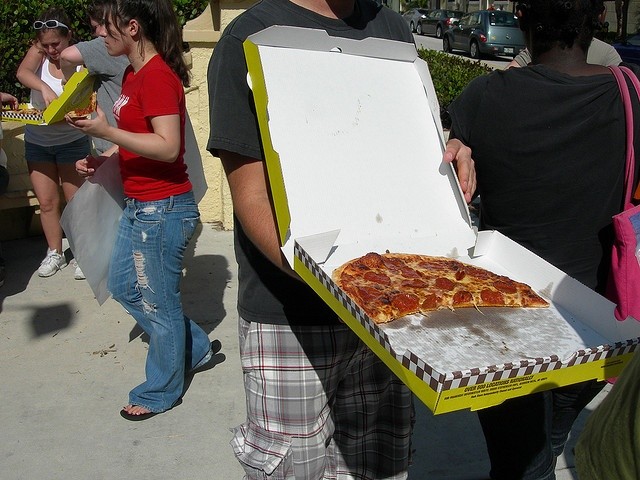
[65,0,220,420]
[503,31,622,70]
[16,14,91,281]
[449,0,640,480]
[1,91,18,202]
[59,1,129,155]
[205,1,477,480]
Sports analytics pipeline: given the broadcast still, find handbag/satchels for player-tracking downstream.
[609,64,640,323]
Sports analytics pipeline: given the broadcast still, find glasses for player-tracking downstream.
[33,20,69,30]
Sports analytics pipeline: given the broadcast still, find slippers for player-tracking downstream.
[120,397,183,421]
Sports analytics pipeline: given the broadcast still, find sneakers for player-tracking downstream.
[37,248,67,277]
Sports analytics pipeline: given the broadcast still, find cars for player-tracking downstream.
[442,10,526,59]
[611,33,640,65]
[401,8,429,33]
[416,9,465,39]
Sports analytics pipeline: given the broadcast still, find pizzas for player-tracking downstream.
[68,91,97,118]
[331,252,550,324]
[1,108,45,116]
